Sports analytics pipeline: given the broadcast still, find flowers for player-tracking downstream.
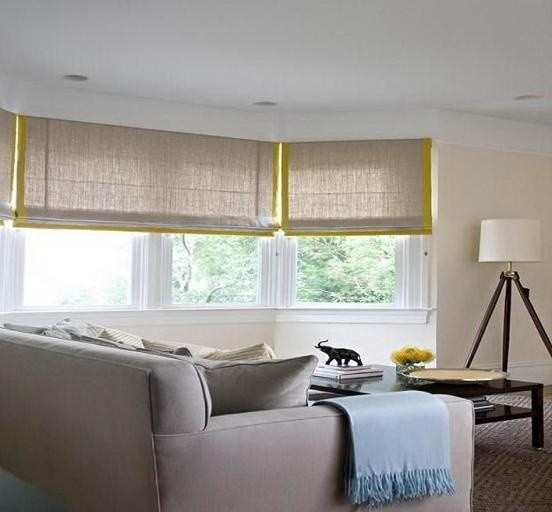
[390,344,436,375]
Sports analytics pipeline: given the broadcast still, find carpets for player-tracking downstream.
[470,384,552,511]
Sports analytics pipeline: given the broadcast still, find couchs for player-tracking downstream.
[1,323,475,512]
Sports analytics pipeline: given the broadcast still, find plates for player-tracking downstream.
[400,368,511,385]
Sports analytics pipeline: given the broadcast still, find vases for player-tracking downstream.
[394,364,426,376]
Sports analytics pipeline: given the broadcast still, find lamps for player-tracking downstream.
[463,218,552,372]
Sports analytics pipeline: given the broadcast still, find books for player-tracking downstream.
[468,395,496,410]
[313,364,383,381]
